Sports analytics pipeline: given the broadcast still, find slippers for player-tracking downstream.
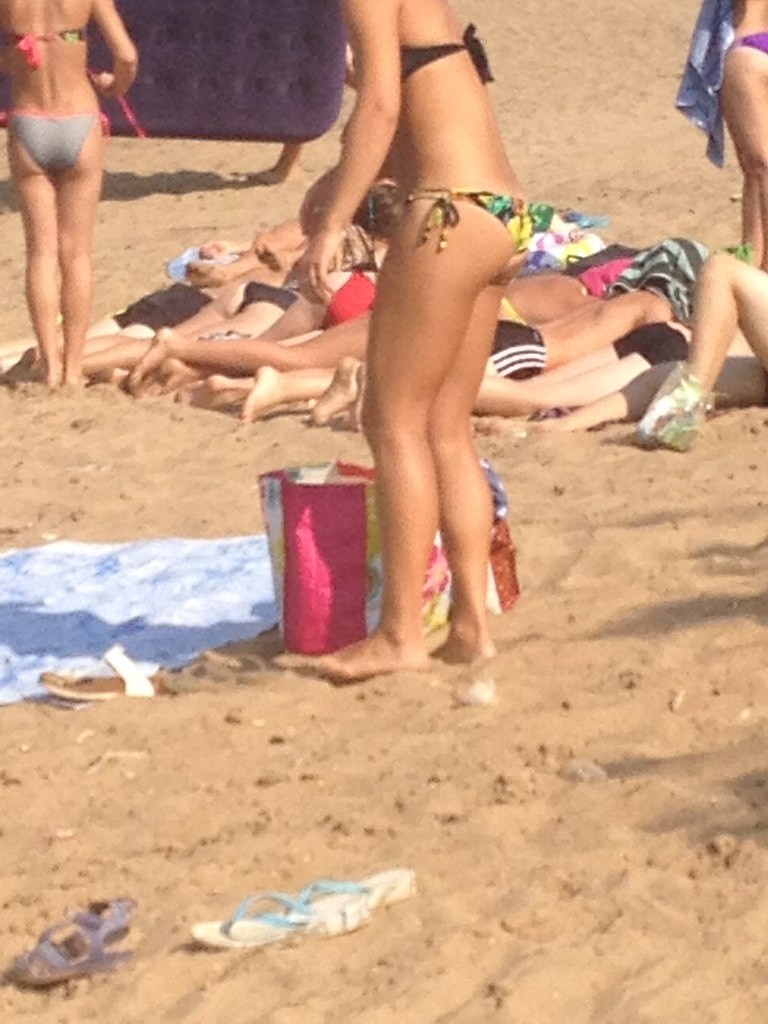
[38,644,166,700]
[10,898,143,985]
[163,657,270,695]
[190,893,359,950]
[282,869,418,919]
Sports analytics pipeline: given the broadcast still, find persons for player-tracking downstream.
[0,203,768,446]
[0,0,140,393]
[297,0,533,686]
[718,0,768,276]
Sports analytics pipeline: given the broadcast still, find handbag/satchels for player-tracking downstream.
[258,459,522,656]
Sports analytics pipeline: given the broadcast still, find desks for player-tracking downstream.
[0,1,347,187]
